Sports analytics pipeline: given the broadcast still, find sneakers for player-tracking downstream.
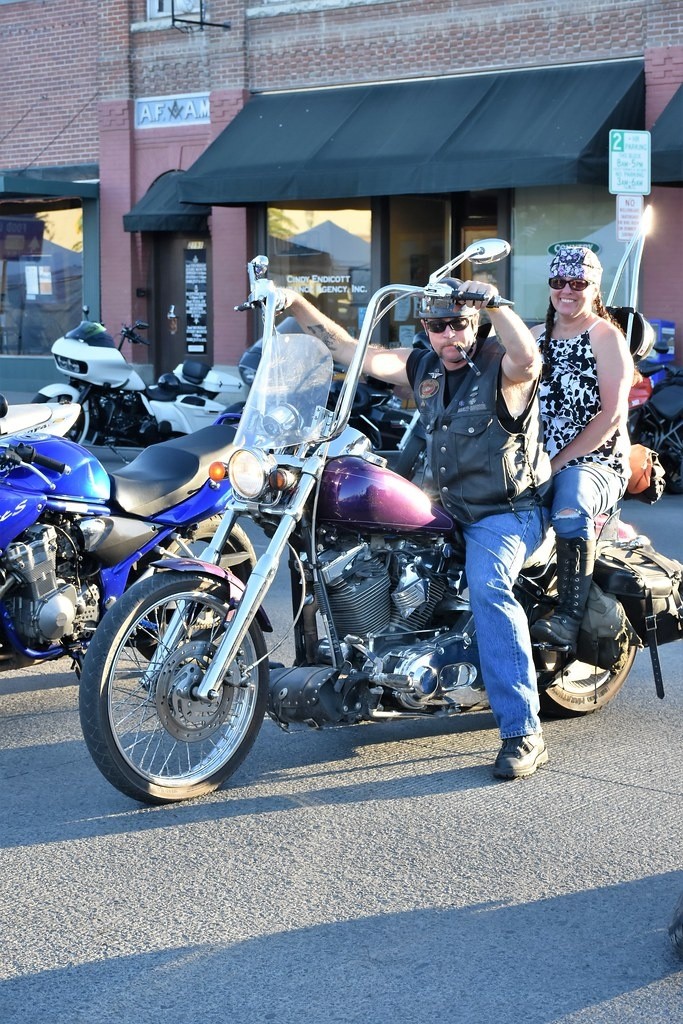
[492,733,548,777]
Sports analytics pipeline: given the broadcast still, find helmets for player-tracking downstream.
[418,277,481,318]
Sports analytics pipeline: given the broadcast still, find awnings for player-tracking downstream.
[0,59,683,234]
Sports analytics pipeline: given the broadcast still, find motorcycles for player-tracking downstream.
[0,238,682,807]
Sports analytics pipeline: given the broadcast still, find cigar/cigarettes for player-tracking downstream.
[455,345,481,377]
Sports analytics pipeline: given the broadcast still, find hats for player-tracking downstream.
[547,246,603,285]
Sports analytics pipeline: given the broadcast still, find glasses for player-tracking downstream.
[548,278,596,291]
[424,315,475,333]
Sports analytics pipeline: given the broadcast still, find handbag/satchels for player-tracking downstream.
[592,541,683,698]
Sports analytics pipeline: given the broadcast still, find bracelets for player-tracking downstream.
[485,308,495,311]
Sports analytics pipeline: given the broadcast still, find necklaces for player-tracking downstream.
[557,325,580,340]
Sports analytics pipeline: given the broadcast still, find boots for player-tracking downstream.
[529,535,596,656]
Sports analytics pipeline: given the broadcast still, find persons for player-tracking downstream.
[275,275,551,778]
[525,246,634,655]
